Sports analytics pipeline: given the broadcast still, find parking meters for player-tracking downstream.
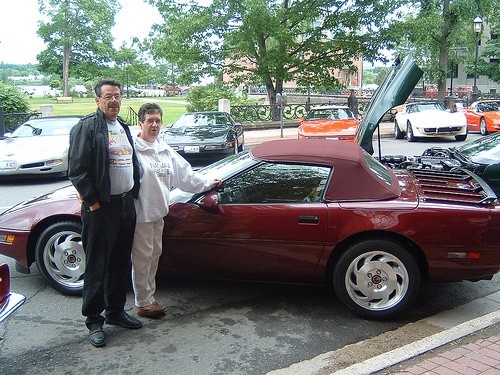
[276,92,287,138]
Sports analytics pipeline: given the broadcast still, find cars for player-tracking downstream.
[121,83,190,97]
[424,83,481,100]
[298,106,361,143]
[0,52,500,321]
[0,115,86,181]
[464,99,500,136]
[70,84,88,94]
[162,111,244,162]
[394,102,468,142]
[422,131,500,202]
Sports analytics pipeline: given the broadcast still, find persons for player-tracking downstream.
[130,103,223,315]
[66,80,142,347]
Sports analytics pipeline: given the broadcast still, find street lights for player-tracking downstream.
[472,17,483,104]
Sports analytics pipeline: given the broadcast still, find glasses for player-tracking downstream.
[99,94,122,101]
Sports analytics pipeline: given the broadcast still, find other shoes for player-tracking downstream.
[138,303,167,317]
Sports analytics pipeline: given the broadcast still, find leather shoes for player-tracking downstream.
[89,327,107,347]
[105,311,142,329]
